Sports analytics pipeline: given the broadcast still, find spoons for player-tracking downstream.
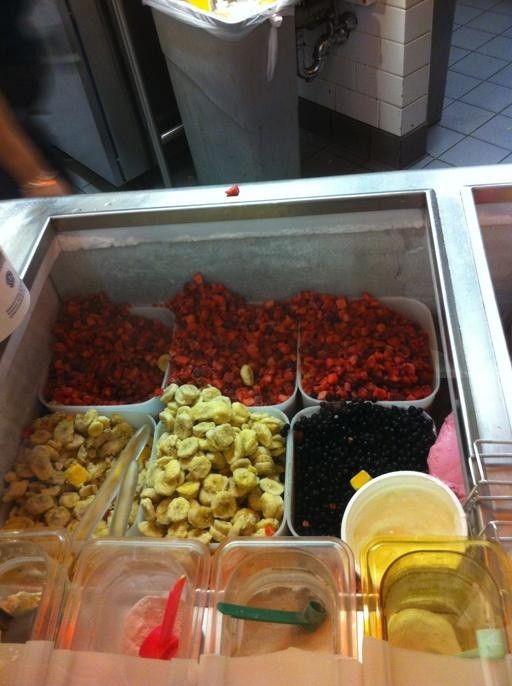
[216,595,326,633]
[136,575,187,658]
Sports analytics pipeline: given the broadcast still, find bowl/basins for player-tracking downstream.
[341,468,470,583]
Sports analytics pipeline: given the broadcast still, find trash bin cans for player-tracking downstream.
[142,0,301,186]
